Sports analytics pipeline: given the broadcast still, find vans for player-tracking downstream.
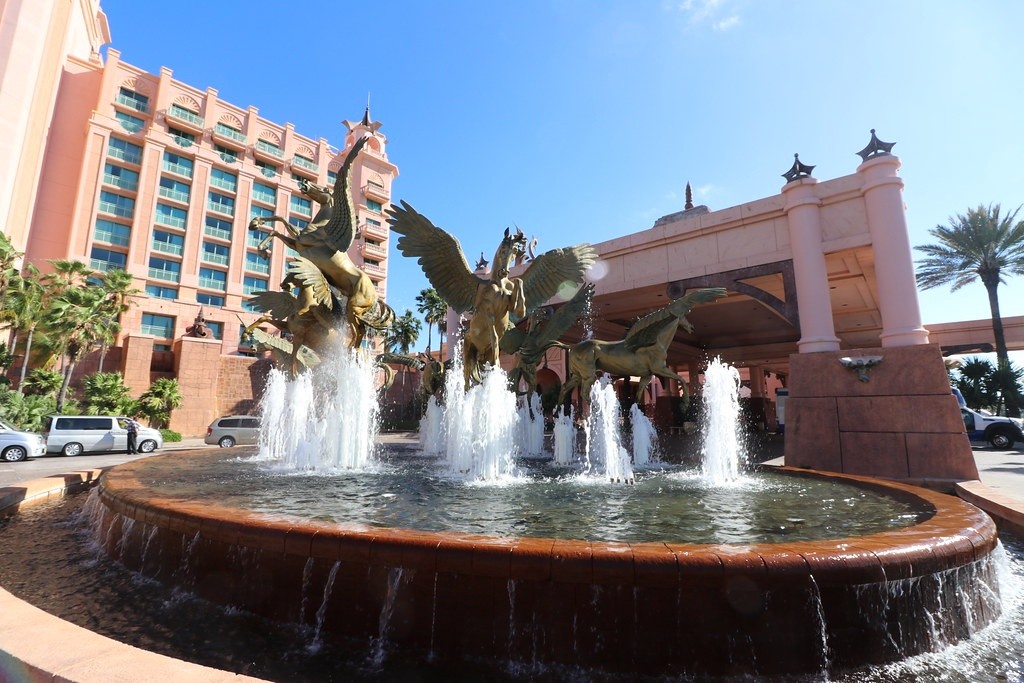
[205,416,270,450]
[41,415,162,455]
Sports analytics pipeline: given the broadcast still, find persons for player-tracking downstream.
[126,416,140,455]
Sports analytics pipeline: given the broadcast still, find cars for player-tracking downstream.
[0,421,46,462]
[951,387,1024,450]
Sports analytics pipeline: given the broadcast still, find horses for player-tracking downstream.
[246,136,728,422]
